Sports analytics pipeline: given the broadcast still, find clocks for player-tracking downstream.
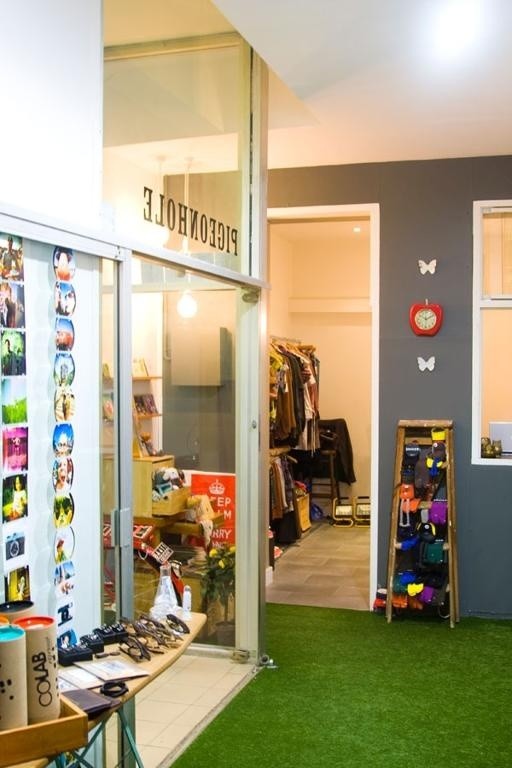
[409,298,443,337]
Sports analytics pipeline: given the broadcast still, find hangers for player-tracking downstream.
[268,335,317,367]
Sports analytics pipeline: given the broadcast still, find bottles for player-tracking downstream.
[0,614,62,732]
[152,563,192,624]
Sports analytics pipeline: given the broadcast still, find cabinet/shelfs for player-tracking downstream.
[268,334,314,562]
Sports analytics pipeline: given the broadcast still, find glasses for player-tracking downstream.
[120,613,190,662]
